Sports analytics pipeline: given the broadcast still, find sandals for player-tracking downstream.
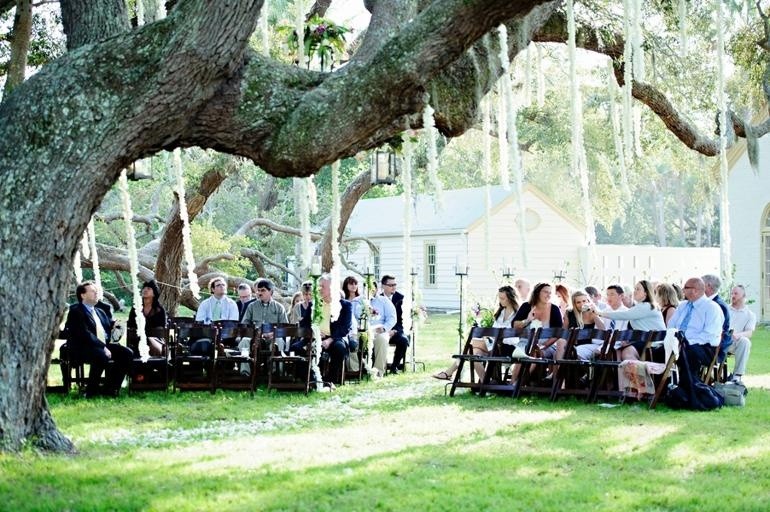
[431,372,452,380]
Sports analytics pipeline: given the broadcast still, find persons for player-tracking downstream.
[126,278,167,384]
[193,275,413,389]
[63,281,135,398]
[432,285,520,396]
[511,275,757,396]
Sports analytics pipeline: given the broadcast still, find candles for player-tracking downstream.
[312,264,320,275]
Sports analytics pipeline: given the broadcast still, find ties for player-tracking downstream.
[678,302,694,334]
[92,309,106,344]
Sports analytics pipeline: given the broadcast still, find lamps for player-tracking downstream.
[370,149,398,185]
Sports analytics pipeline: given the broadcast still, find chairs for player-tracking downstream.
[448,323,734,409]
